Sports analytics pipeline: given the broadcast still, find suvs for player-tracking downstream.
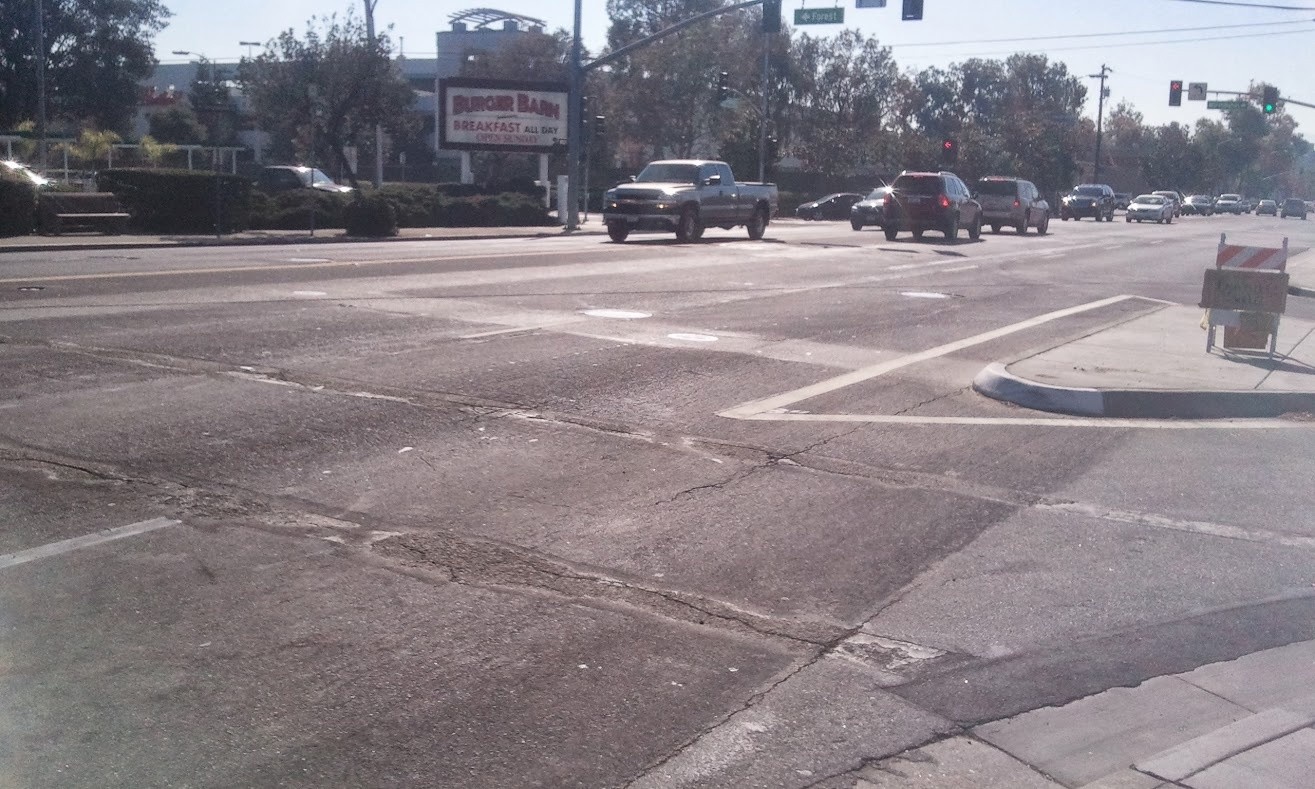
[973,176,1050,235]
[1061,184,1115,222]
[883,170,986,241]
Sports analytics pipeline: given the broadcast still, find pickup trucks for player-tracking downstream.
[603,159,779,243]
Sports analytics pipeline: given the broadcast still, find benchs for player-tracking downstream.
[36,191,131,235]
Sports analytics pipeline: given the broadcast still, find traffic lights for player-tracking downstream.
[1169,81,1183,106]
[902,0,924,21]
[944,138,957,161]
[762,0,782,34]
[1263,86,1277,114]
[595,115,606,139]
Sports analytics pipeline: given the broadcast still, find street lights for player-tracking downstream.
[239,41,260,63]
[172,50,221,238]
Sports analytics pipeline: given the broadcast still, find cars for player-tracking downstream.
[1181,193,1252,216]
[0,160,98,204]
[794,186,890,230]
[1116,191,1181,224]
[264,165,355,208]
[1256,198,1315,220]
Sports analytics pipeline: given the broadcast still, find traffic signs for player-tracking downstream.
[1188,83,1207,99]
[794,8,845,24]
